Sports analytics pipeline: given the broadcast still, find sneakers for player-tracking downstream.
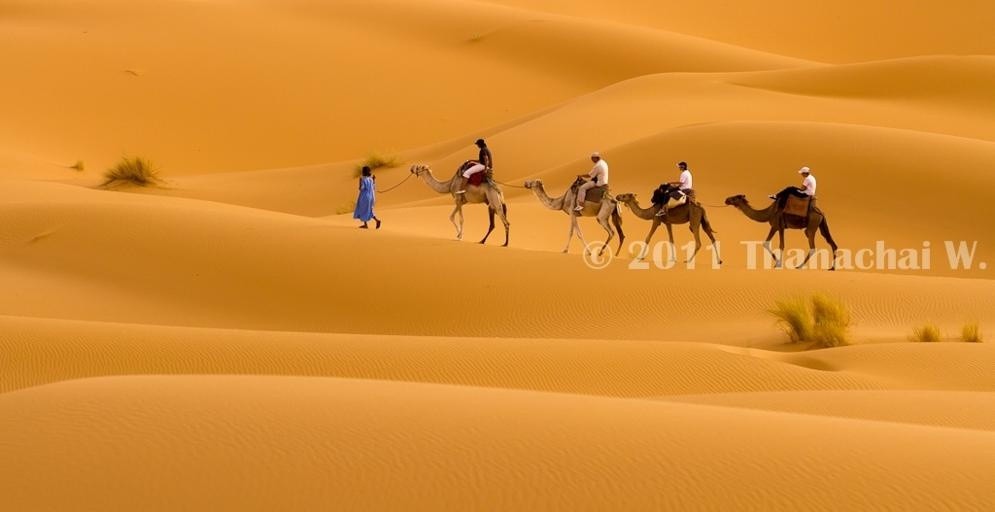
[574,207,584,210]
[455,190,466,194]
[656,210,666,216]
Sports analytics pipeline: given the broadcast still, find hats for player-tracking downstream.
[475,139,486,146]
[590,152,599,157]
[799,167,810,173]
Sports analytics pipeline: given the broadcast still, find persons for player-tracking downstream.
[768,166,817,214]
[574,153,609,212]
[353,165,381,229]
[455,138,493,195]
[655,162,694,217]
